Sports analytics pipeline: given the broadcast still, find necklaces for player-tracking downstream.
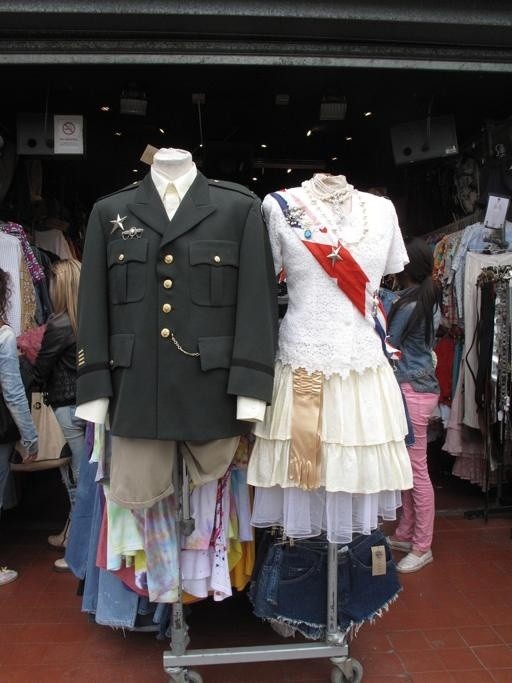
[301,176,371,244]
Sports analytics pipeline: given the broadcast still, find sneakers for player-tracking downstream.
[0,568,18,586]
[386,536,412,553]
[395,550,434,573]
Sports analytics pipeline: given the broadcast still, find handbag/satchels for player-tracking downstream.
[9,392,72,471]
[1,319,32,444]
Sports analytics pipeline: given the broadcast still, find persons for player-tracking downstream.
[249,165,417,540]
[73,143,280,509]
[17,257,87,572]
[378,235,448,575]
[2,265,40,584]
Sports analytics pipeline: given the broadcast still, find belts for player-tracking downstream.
[466,265,512,472]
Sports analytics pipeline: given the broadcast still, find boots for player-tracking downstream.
[47,518,71,548]
[53,558,70,573]
